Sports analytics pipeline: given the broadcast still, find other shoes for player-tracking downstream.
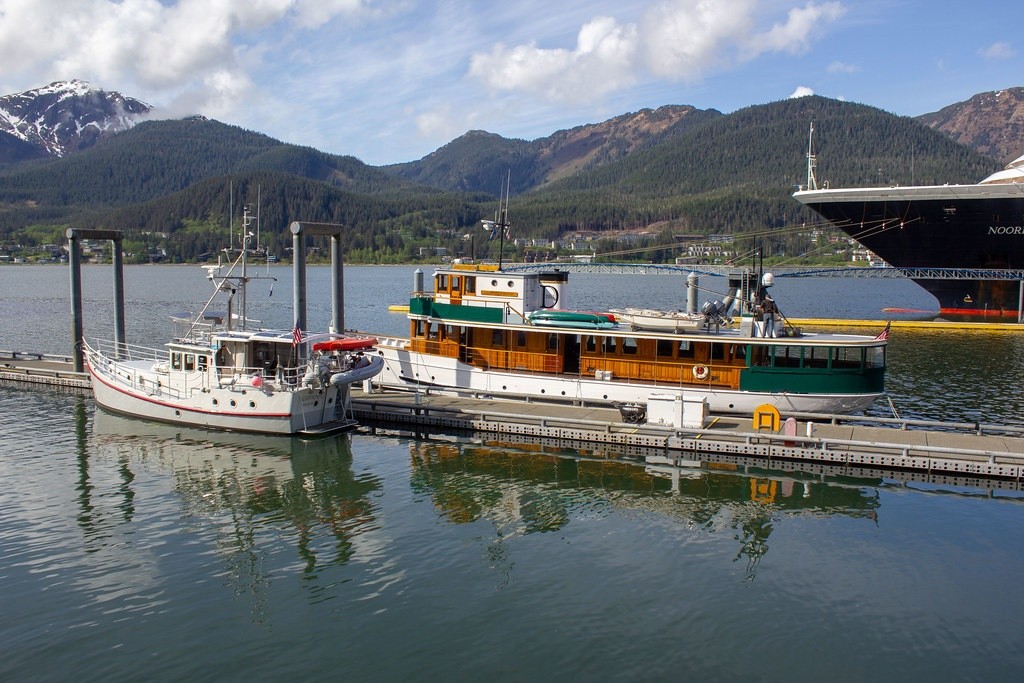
[769,336,773,338]
[762,336,765,338]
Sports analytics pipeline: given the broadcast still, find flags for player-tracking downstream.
[269,283,273,296]
[292,320,303,347]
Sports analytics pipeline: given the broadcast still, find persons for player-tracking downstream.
[760,294,781,338]
[216,344,226,372]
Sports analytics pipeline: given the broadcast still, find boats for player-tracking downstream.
[793,120,1024,316]
[91,403,381,573]
[74,177,385,440]
[333,167,891,419]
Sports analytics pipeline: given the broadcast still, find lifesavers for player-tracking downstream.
[692,363,709,379]
